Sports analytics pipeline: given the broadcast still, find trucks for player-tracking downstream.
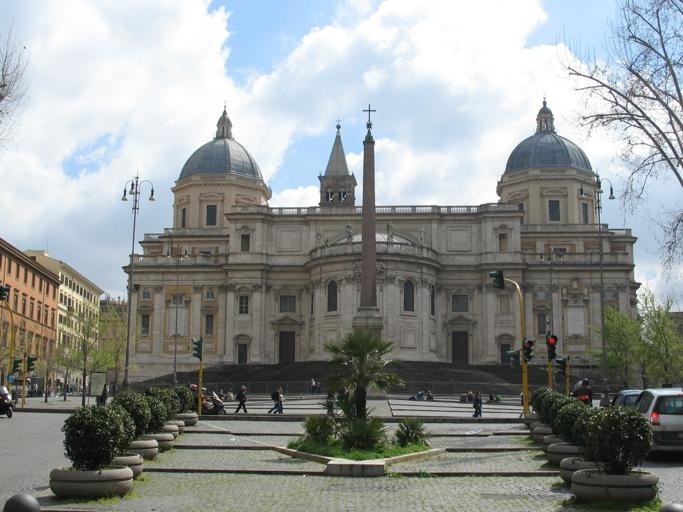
[11,377,43,398]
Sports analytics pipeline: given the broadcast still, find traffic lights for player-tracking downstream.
[27,355,38,371]
[522,336,535,363]
[12,358,21,373]
[0,284,11,302]
[506,350,520,367]
[486,269,505,290]
[554,358,568,376]
[191,336,202,360]
[546,333,558,360]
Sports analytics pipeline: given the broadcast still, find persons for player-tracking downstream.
[519,378,592,407]
[190,377,322,415]
[408,388,436,401]
[460,389,501,418]
[26,381,112,413]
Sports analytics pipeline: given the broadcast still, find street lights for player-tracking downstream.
[120,172,156,390]
[576,166,618,388]
[161,243,188,384]
[538,247,564,333]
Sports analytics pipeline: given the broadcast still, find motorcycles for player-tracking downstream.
[190,390,226,414]
[0,385,15,419]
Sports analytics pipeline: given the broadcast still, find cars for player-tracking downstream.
[600,384,683,460]
